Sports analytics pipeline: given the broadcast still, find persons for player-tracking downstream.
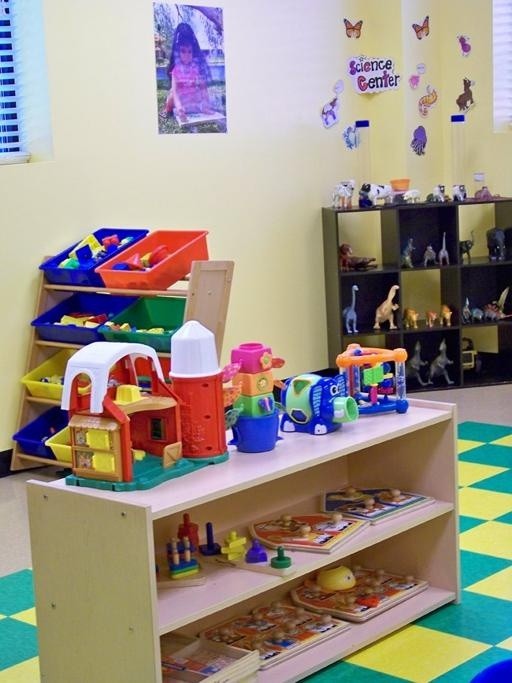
[160,20,216,125]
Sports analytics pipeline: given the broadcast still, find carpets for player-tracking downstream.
[0,419,512,683]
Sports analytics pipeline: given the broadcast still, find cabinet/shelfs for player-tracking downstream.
[26,392,461,683]
[321,194,512,393]
[10,255,234,469]
